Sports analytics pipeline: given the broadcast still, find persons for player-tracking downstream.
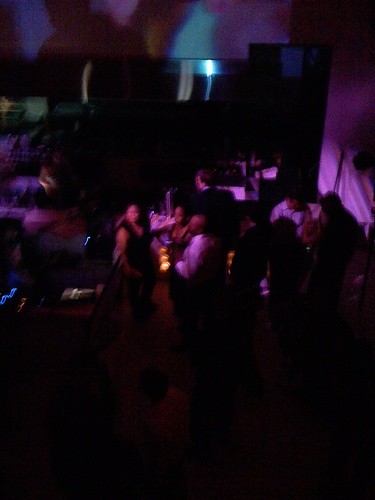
[318,191,368,285]
[285,186,314,248]
[38,147,87,211]
[113,202,160,328]
[164,202,194,266]
[168,214,223,317]
[190,168,240,256]
